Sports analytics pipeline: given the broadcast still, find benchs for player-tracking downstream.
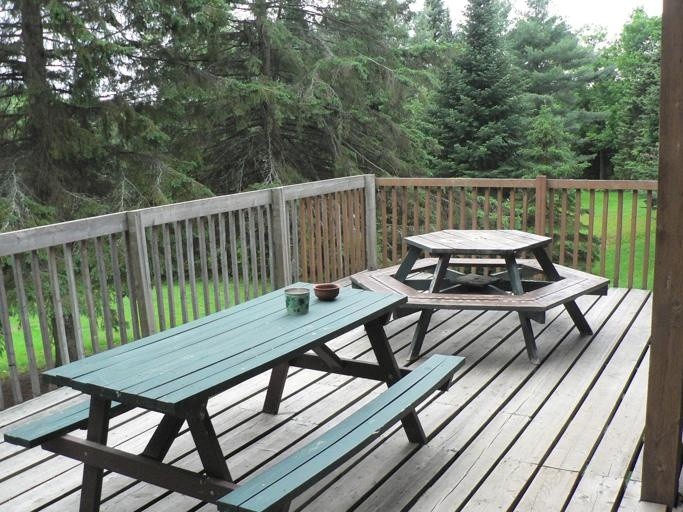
[347,257,610,324]
[215,353,468,511]
[4,394,137,458]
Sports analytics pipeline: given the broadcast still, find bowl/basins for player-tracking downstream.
[314,283,340,301]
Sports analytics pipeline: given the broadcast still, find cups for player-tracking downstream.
[284,288,310,315]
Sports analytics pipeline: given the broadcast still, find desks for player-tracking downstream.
[38,281,429,512]
[370,230,595,367]
